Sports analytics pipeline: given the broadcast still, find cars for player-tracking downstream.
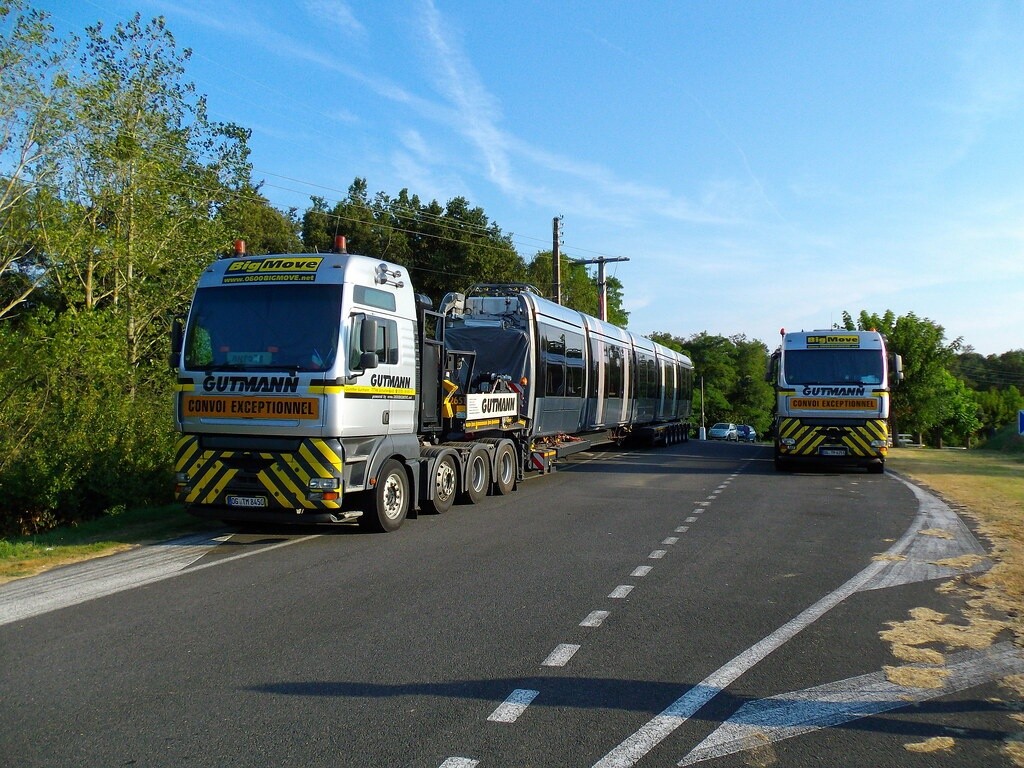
[707,422,739,442]
[737,425,757,443]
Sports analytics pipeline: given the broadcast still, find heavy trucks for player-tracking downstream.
[766,327,904,474]
[166,236,694,534]
